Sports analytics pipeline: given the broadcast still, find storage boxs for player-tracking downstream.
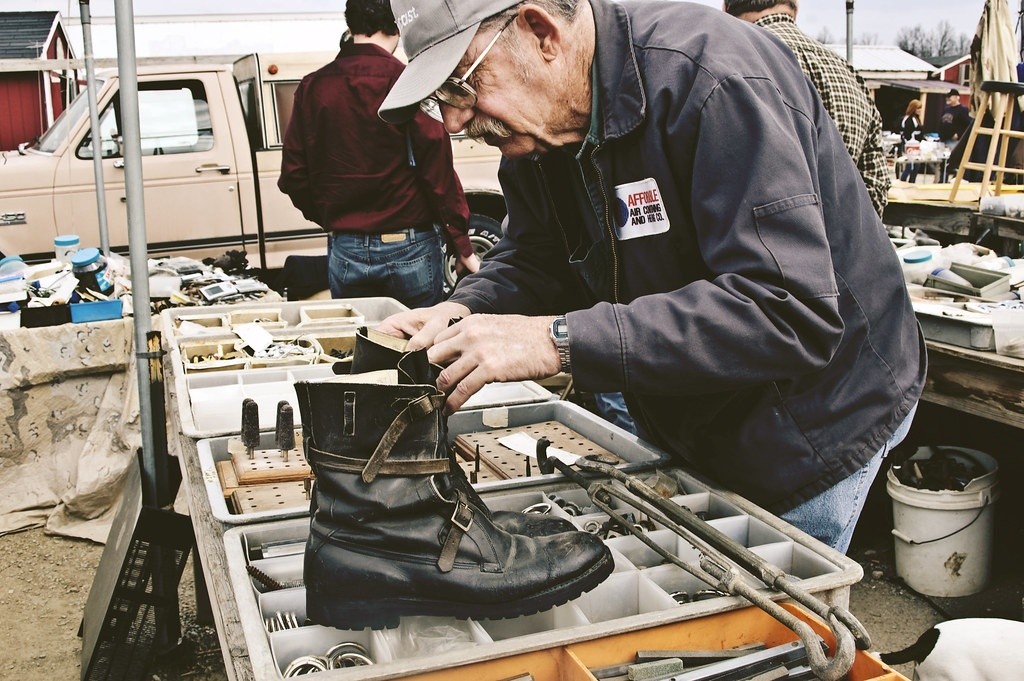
[18,296,1024,678]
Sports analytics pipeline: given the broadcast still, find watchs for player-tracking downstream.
[550,311,575,373]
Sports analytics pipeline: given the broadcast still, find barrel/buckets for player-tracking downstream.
[885,445,1001,597]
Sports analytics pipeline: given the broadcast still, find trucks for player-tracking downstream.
[1,44,507,297]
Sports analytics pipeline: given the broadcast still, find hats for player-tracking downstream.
[378,0,530,125]
[945,89,959,97]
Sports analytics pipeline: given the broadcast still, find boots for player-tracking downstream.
[329,326,578,540]
[292,380,614,631]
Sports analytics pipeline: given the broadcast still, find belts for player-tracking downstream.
[333,222,432,239]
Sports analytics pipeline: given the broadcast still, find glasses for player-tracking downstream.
[418,12,520,123]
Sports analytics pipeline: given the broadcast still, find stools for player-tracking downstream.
[947,81,1024,212]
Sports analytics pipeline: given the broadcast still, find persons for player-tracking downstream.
[937,88,971,183]
[895,98,925,183]
[278,0,484,308]
[356,0,934,575]
[718,1,894,224]
